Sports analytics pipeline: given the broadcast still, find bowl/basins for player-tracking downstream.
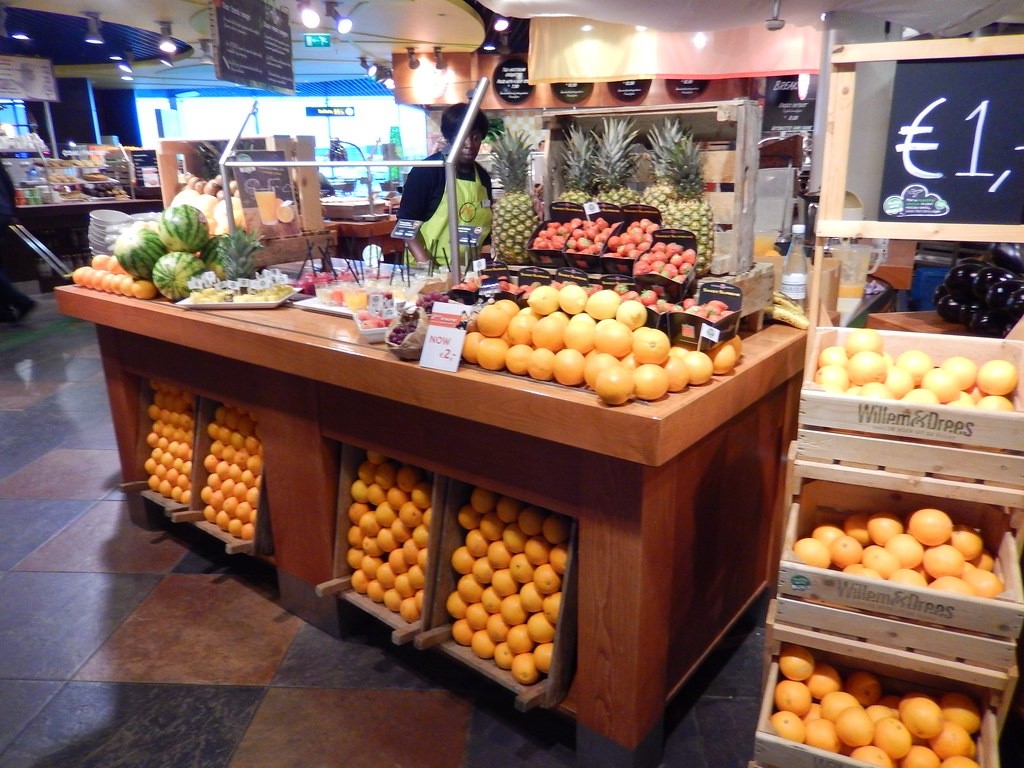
[353,309,401,344]
[88,209,162,257]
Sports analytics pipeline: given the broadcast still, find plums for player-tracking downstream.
[932,258,1024,337]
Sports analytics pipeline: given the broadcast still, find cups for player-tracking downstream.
[300,265,472,313]
[753,232,781,256]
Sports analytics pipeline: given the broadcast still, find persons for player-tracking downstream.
[392,101,495,270]
[0,160,38,321]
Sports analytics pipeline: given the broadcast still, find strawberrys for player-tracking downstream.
[451,218,734,323]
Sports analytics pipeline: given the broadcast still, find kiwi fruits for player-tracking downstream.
[187,175,242,199]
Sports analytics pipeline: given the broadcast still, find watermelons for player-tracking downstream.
[113,205,232,301]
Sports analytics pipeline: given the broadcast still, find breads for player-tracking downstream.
[34,160,130,199]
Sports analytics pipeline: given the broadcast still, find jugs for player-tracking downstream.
[829,243,883,299]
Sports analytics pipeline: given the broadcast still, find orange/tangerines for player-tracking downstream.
[145,378,263,540]
[447,489,568,684]
[769,646,980,768]
[791,507,1003,630]
[172,189,294,236]
[813,329,1018,454]
[347,451,432,624]
[462,284,743,405]
[72,255,157,300]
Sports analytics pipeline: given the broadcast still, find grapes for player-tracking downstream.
[389,292,467,345]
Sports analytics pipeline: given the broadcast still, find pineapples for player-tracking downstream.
[487,127,539,266]
[558,115,715,277]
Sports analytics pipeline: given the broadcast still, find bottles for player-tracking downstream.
[30,227,92,294]
[781,224,807,311]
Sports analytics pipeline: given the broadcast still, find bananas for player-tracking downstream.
[773,291,810,329]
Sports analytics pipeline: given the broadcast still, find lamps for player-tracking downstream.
[430,51,448,70]
[83,17,105,46]
[157,26,178,55]
[480,14,516,58]
[120,74,133,85]
[117,54,134,73]
[106,54,123,61]
[359,58,396,90]
[403,51,421,69]
[160,56,174,69]
[329,11,355,37]
[7,24,31,43]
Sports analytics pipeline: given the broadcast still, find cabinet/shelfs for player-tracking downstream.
[748,226,1024,767]
[3,143,161,210]
[543,96,765,289]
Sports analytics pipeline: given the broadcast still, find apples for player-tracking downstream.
[16,190,41,205]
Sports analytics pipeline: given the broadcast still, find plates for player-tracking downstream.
[174,287,302,308]
[294,296,413,318]
